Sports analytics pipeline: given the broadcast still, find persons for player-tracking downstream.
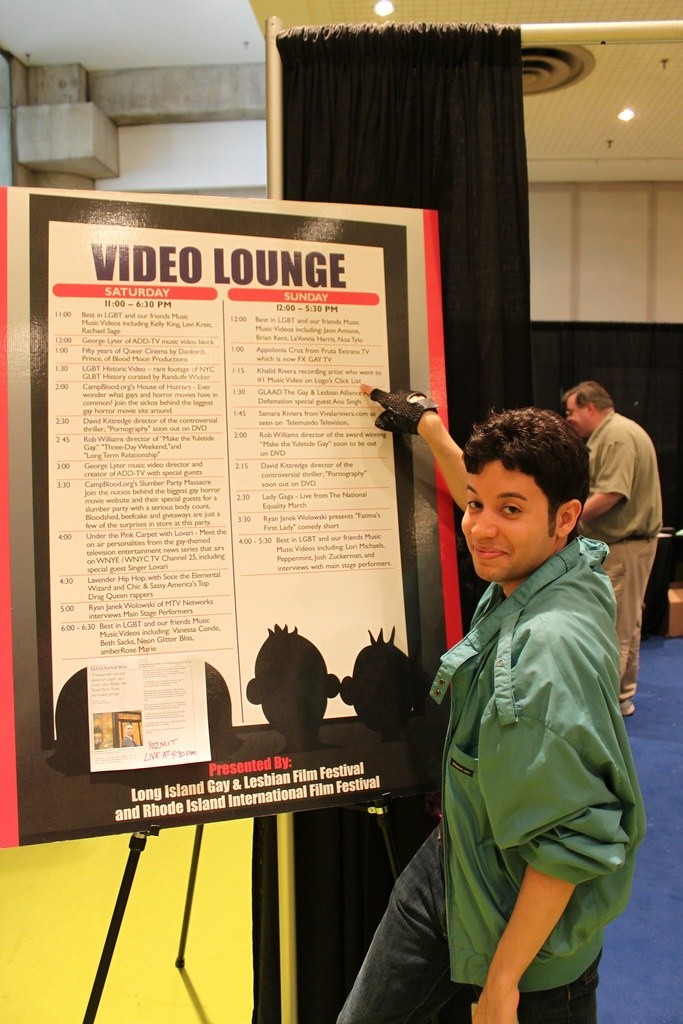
[563,381,662,717]
[336,384,646,1024]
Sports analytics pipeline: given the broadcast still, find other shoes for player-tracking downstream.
[621,700,635,716]
